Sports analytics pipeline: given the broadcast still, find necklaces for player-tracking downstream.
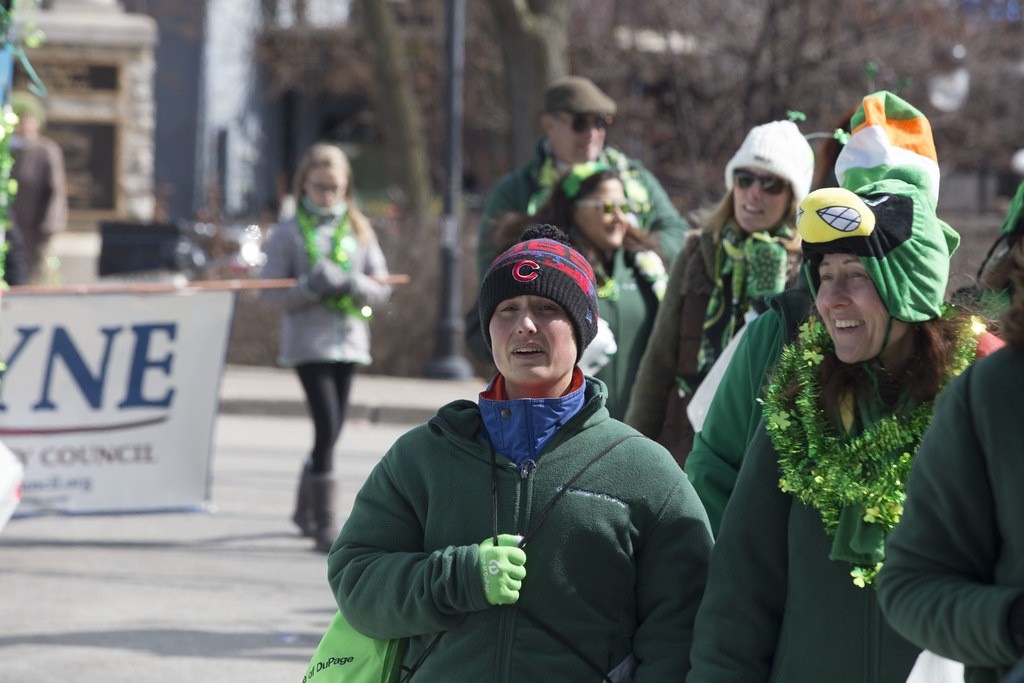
[299,210,351,308]
[761,301,976,586]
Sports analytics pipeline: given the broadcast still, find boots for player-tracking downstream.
[311,471,340,551]
[292,465,333,536]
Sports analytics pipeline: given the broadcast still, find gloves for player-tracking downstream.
[906,650,965,683]
[307,258,343,294]
[478,534,526,604]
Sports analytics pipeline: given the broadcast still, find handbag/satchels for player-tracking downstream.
[302,610,404,683]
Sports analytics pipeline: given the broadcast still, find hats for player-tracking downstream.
[479,223,598,368]
[796,90,960,323]
[543,76,617,115]
[725,120,814,209]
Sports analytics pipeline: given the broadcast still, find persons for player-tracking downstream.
[462,164,668,422]
[624,92,1024,683]
[4,91,68,287]
[480,77,692,268]
[325,222,716,683]
[256,142,390,548]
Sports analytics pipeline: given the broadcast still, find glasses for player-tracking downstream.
[732,170,785,194]
[574,198,633,215]
[308,178,345,199]
[554,115,608,131]
